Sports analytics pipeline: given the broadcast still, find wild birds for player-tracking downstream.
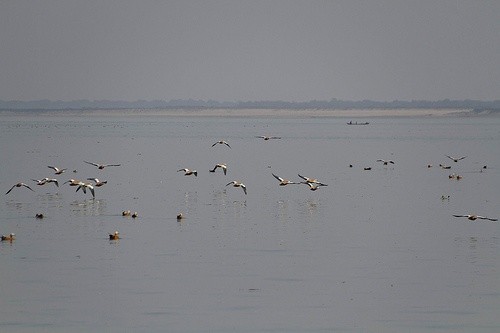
[256,135,282,141]
[225,181,247,195]
[35,214,43,219]
[348,159,395,171]
[209,164,227,175]
[6,161,121,197]
[122,210,138,218]
[347,121,370,125]
[109,232,119,240]
[453,213,499,223]
[211,141,231,148]
[177,214,183,220]
[0,233,16,241]
[177,168,197,177]
[272,172,329,191]
[426,151,490,181]
